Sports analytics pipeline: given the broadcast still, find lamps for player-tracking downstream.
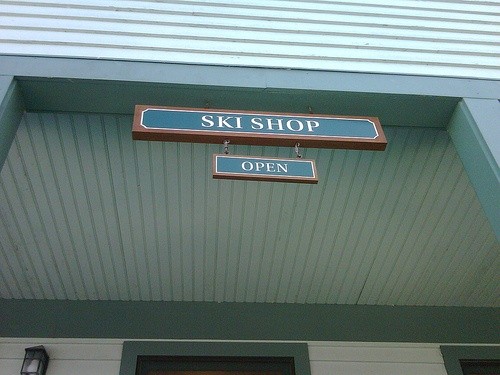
[20,345,50,375]
